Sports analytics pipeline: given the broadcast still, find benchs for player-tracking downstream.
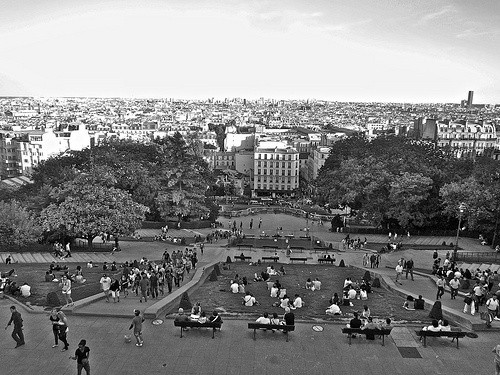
[290,257,307,263]
[260,236,269,238]
[234,255,251,262]
[247,321,295,342]
[342,326,391,345]
[318,258,336,264]
[174,318,222,338]
[263,245,278,252]
[300,236,310,240]
[262,256,279,262]
[285,235,295,239]
[314,247,329,253]
[290,246,305,253]
[272,236,282,239]
[237,244,253,250]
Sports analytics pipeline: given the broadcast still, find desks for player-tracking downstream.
[415,329,467,349]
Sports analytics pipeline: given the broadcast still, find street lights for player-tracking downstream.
[452,202,466,264]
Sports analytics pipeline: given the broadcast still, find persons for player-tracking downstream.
[0,241,93,307]
[249,259,261,266]
[329,277,393,339]
[49,308,69,352]
[287,245,291,256]
[273,253,278,262]
[230,264,321,333]
[479,234,488,245]
[319,253,335,264]
[175,301,224,332]
[342,231,416,285]
[152,217,262,254]
[274,225,294,241]
[403,245,500,332]
[69,339,91,375]
[311,219,323,227]
[5,306,25,348]
[240,253,245,261]
[99,248,198,303]
[128,309,145,347]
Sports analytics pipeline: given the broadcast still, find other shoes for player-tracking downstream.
[15,341,25,348]
[52,345,59,347]
[61,349,66,352]
[136,343,143,347]
